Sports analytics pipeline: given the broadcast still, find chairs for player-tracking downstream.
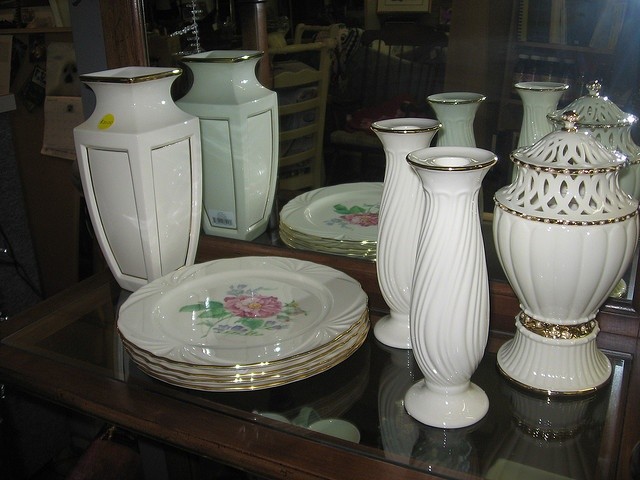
[265,35,339,230]
[294,22,340,43]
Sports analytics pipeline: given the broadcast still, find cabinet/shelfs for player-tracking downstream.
[491,0,628,206]
[0,234,640,480]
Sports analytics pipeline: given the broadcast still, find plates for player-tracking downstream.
[279,182,386,260]
[116,255,369,391]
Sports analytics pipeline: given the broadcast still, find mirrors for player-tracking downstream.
[99,0,640,353]
